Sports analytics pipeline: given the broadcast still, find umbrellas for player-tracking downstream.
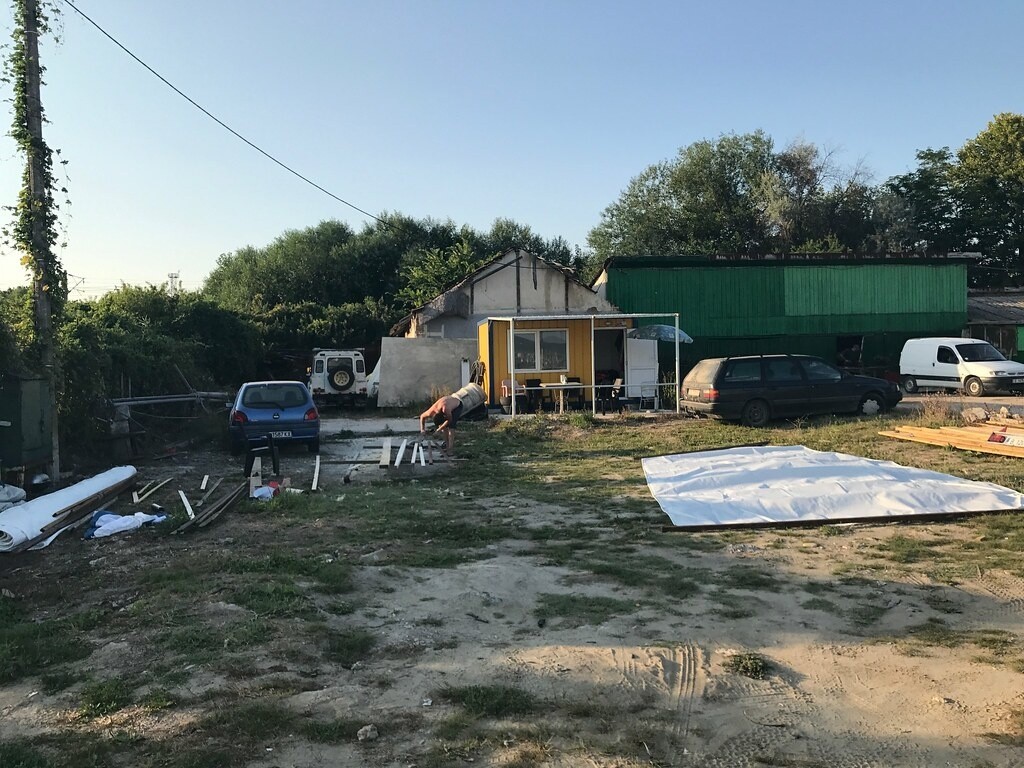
[625,323,693,386]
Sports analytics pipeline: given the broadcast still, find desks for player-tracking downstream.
[539,382,582,414]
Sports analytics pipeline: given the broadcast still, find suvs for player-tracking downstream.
[899,337,1024,397]
[306,347,367,409]
[679,355,902,428]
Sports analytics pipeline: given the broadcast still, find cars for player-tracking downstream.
[225,381,319,452]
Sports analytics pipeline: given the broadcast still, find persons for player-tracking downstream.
[421,396,463,449]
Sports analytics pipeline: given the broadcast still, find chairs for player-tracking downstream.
[502,377,659,412]
